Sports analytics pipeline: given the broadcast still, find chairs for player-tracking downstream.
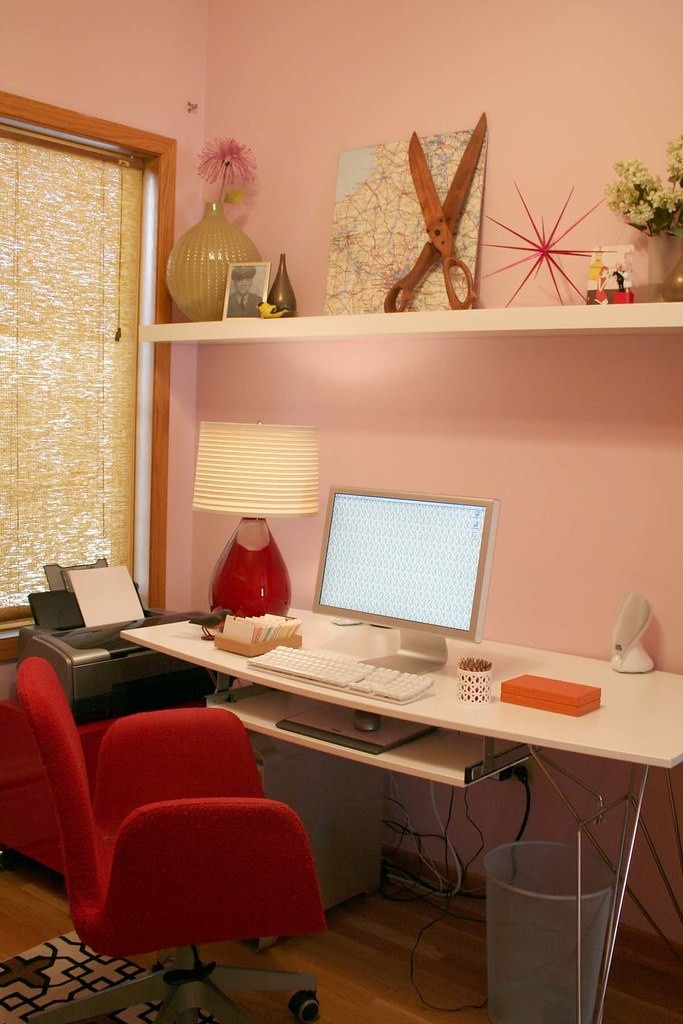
[18,657,325,1024]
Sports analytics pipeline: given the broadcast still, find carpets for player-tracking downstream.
[0,933,225,1024]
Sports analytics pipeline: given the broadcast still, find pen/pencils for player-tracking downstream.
[456,657,493,704]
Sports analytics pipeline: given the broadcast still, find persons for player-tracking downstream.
[227,266,263,317]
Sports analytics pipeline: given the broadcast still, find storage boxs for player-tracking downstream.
[212,632,301,658]
[501,675,601,719]
[613,292,634,303]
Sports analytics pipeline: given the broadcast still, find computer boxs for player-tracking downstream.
[250,728,382,912]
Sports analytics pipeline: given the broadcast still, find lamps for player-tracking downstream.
[191,420,320,629]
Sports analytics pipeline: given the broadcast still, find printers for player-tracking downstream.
[17,557,237,725]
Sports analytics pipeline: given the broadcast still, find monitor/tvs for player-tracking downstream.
[311,485,501,676]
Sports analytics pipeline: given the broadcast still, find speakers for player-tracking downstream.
[609,593,654,673]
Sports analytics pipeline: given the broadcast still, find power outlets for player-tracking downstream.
[512,762,536,783]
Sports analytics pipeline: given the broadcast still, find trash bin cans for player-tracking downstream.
[483,836,615,1024]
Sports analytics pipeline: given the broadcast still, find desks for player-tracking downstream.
[120,607,683,1024]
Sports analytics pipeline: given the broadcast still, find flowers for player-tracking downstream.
[606,135,683,237]
[199,135,252,204]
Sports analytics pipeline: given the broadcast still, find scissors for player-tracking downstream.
[379,112,492,313]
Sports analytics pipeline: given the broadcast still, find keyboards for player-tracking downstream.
[246,645,437,705]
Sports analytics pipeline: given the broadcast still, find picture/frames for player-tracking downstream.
[222,261,272,323]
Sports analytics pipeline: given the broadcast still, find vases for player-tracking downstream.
[648,236,683,303]
[166,204,260,321]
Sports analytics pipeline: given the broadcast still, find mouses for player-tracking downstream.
[354,710,380,732]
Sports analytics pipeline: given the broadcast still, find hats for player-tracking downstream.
[231,266,256,280]
